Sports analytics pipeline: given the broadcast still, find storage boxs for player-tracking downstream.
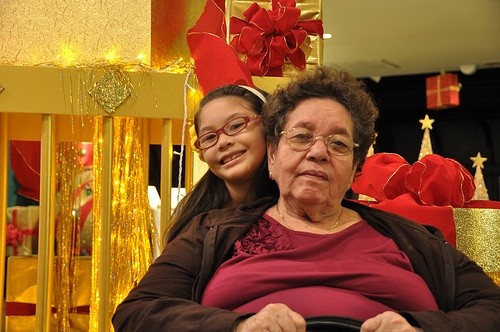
[7,207,33,257]
[427,73,460,110]
[225,0,324,77]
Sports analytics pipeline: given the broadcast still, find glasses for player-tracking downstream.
[278,127,360,156]
[193,114,263,150]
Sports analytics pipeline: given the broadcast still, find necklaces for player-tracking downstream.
[277,205,341,232]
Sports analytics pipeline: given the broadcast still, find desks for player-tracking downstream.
[4,254,92,332]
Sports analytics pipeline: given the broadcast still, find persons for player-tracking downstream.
[162,82,272,244]
[110,64,500,332]
